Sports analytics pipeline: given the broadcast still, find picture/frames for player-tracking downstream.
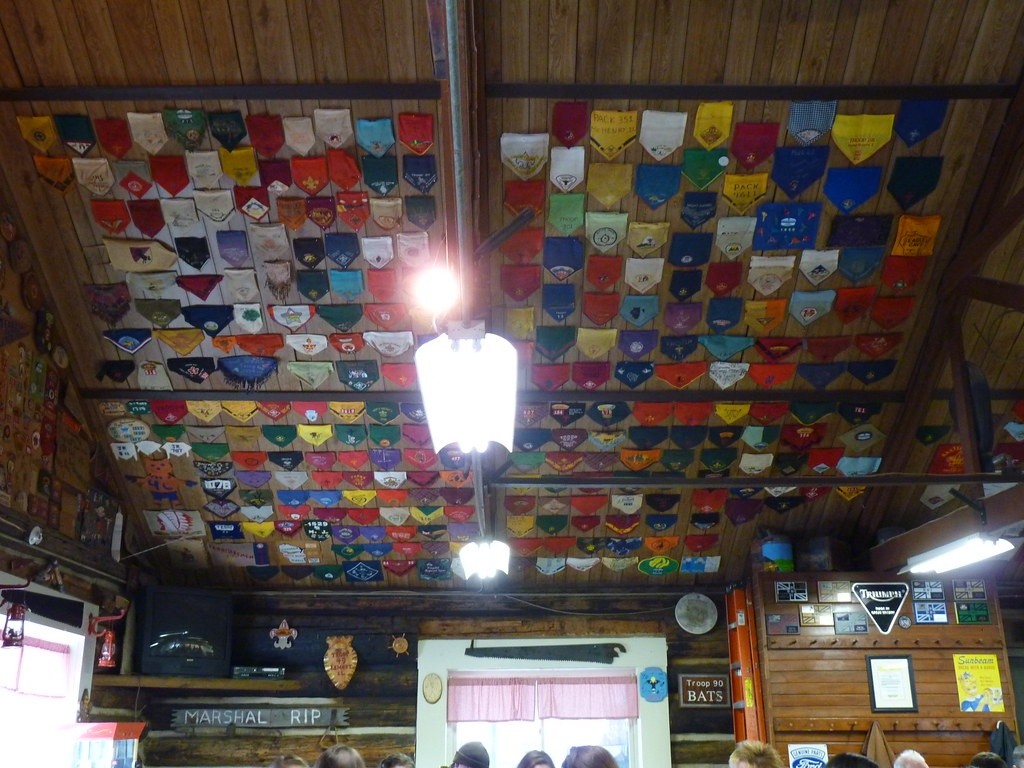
[865,654,919,713]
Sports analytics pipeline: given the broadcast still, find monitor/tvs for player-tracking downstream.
[138,586,233,677]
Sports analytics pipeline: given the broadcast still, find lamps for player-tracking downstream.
[0,574,32,649]
[414,0,517,454]
[88,609,126,667]
[896,533,1014,576]
[458,451,510,580]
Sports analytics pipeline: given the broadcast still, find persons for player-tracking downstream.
[729,740,786,768]
[313,744,366,768]
[268,754,310,768]
[893,749,930,768]
[1012,744,1024,768]
[437,742,490,768]
[566,745,619,768]
[967,751,1008,768]
[379,752,416,768]
[516,750,555,768]
